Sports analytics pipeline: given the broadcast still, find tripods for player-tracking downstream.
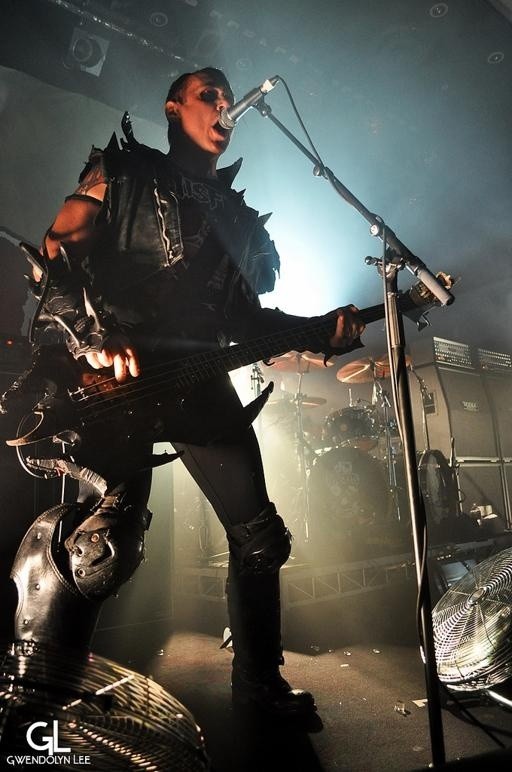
[200,366,408,572]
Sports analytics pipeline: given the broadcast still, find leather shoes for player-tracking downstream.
[232,678,318,716]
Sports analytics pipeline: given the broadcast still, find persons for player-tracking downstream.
[0,69,365,738]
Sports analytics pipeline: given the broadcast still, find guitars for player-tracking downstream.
[5,269,462,447]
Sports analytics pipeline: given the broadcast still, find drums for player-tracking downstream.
[323,405,380,453]
[304,447,392,545]
[377,413,404,451]
[390,451,456,531]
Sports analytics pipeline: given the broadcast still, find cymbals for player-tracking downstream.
[336,347,413,385]
[262,395,327,418]
[262,350,337,374]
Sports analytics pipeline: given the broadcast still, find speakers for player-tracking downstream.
[447,464,510,535]
[481,371,512,463]
[407,364,502,463]
[502,463,512,531]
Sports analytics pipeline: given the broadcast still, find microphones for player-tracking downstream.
[219,77,279,130]
